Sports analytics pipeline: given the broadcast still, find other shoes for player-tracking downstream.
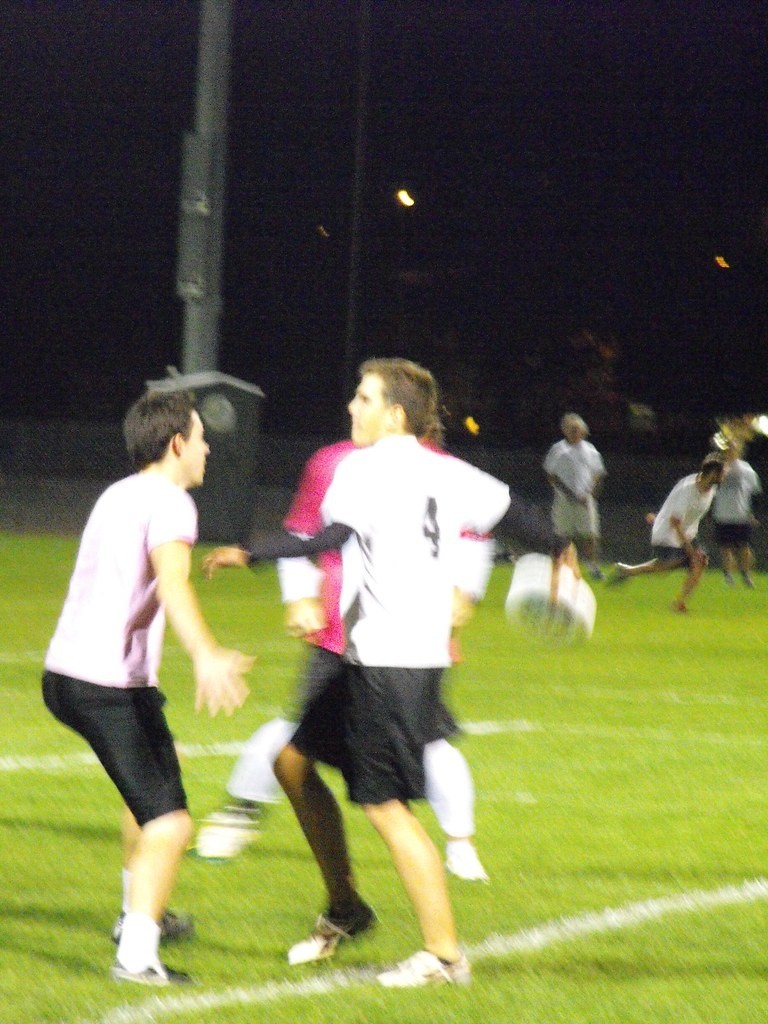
[447,842,489,883]
[196,807,258,859]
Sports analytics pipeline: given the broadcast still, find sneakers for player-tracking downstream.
[114,906,195,951]
[286,896,378,967]
[381,949,474,993]
[113,957,190,989]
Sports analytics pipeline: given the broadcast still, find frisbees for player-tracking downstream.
[506,552,598,639]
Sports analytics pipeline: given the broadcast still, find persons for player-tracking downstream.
[611,446,763,611]
[41,392,252,986]
[546,415,603,579]
[196,357,510,987]
